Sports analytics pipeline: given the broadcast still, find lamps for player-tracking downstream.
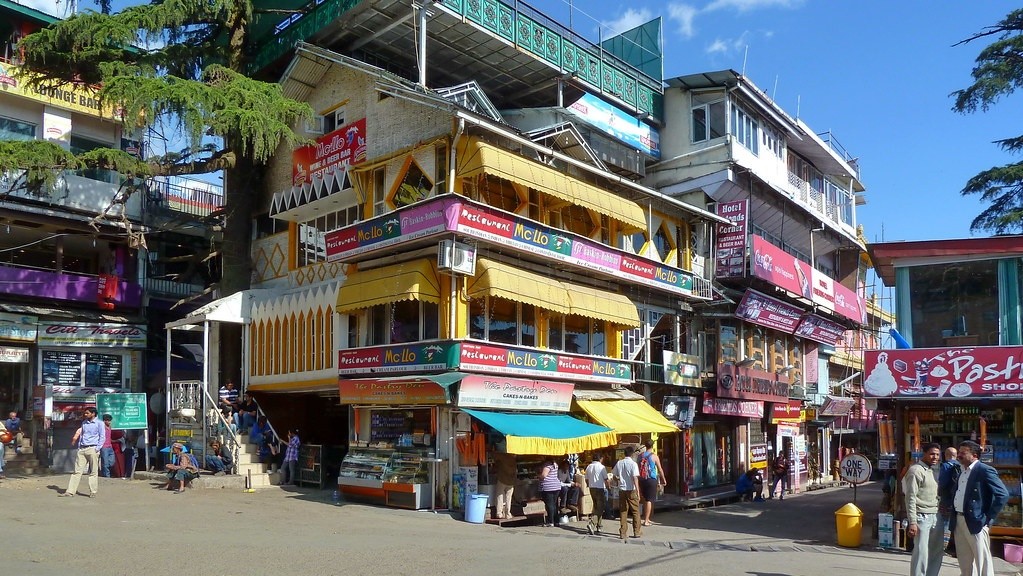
[734,358,756,366]
[777,365,795,375]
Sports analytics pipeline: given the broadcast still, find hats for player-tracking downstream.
[173,443,183,450]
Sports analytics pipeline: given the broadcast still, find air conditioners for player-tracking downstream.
[437,238,474,273]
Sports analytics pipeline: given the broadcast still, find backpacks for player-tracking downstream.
[639,451,653,477]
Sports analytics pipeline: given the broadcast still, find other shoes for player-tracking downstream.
[544,522,554,527]
[9,444,13,448]
[276,468,283,474]
[59,492,73,497]
[644,520,654,526]
[215,471,225,477]
[278,481,285,485]
[174,488,186,494]
[588,523,596,534]
[267,470,273,474]
[89,492,96,498]
[561,508,571,514]
[16,449,21,454]
[569,505,577,510]
[160,486,174,490]
[287,482,293,485]
[598,529,604,535]
[498,514,513,519]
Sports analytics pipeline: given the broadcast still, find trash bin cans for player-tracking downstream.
[465,493,489,524]
[834,502,864,547]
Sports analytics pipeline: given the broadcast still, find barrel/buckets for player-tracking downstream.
[1003,543,1023,563]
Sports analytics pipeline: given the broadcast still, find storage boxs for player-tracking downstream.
[459,465,480,496]
[878,513,895,546]
[576,474,620,513]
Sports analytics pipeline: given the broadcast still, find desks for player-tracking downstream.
[943,334,981,346]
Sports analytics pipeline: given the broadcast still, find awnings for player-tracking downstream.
[460,408,618,456]
[576,399,683,434]
[336,258,440,317]
[457,134,648,236]
[468,258,640,331]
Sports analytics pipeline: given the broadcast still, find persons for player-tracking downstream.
[537,459,561,527]
[205,440,233,476]
[558,460,582,512]
[100,414,115,478]
[218,378,300,485]
[58,407,105,498]
[0,420,9,478]
[736,468,766,502]
[902,441,1010,576]
[766,450,788,501]
[585,453,611,535]
[637,439,667,527]
[611,447,641,539]
[6,412,24,456]
[492,452,517,519]
[159,442,200,494]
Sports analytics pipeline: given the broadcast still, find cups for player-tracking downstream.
[916,368,928,386]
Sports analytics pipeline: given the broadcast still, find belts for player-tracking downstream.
[81,444,97,449]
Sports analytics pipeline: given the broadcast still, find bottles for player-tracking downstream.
[943,407,1021,497]
[794,259,810,297]
[399,433,413,446]
[666,361,699,379]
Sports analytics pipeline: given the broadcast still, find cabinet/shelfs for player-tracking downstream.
[487,451,543,484]
[903,406,1023,537]
[385,448,431,484]
[371,425,411,440]
[341,449,395,479]
